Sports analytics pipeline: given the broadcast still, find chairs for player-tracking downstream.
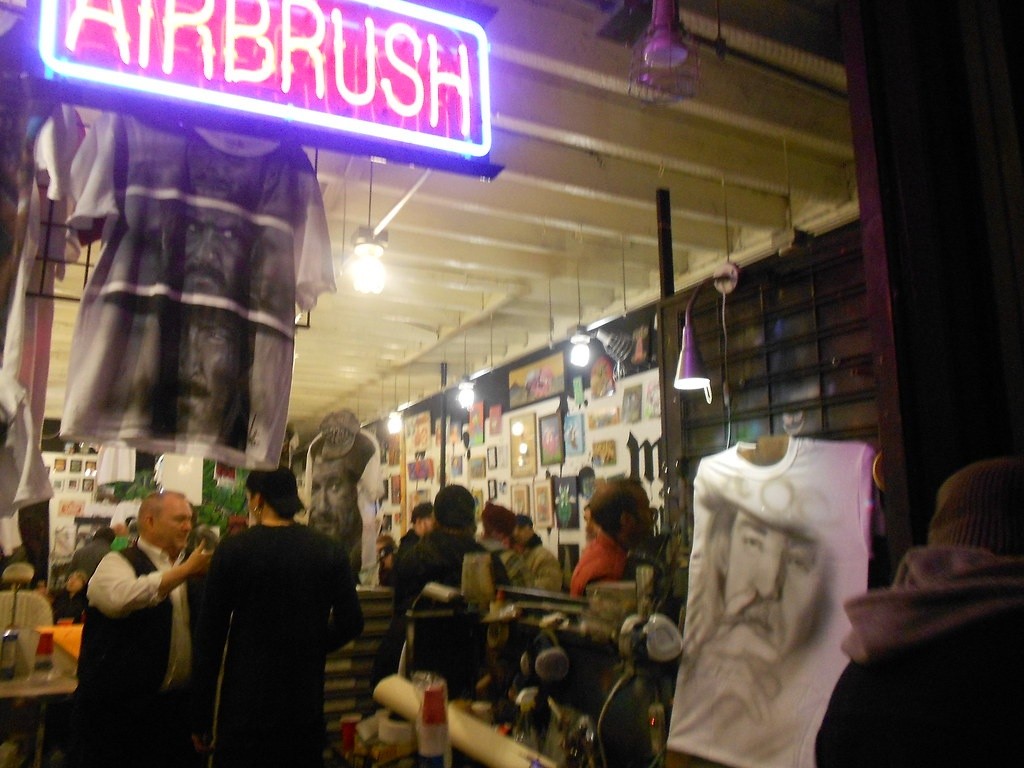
[0,590,57,630]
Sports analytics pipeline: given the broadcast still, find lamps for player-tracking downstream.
[674,262,738,390]
[628,1,697,109]
[388,370,402,433]
[457,331,474,408]
[570,261,591,368]
[347,161,387,293]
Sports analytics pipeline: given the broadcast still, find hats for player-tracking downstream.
[482,503,517,537]
[246,463,305,516]
[435,484,476,529]
[929,453,1024,558]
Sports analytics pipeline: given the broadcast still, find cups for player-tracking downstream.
[341,721,356,748]
[37,634,54,654]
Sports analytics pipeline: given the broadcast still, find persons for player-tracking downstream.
[62,492,212,768]
[46,485,651,726]
[815,457,1024,768]
[189,464,363,768]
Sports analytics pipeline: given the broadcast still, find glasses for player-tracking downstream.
[632,506,658,525]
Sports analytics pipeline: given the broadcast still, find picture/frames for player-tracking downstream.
[54,459,96,491]
[376,356,643,589]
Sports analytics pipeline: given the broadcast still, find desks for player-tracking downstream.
[0,622,84,768]
